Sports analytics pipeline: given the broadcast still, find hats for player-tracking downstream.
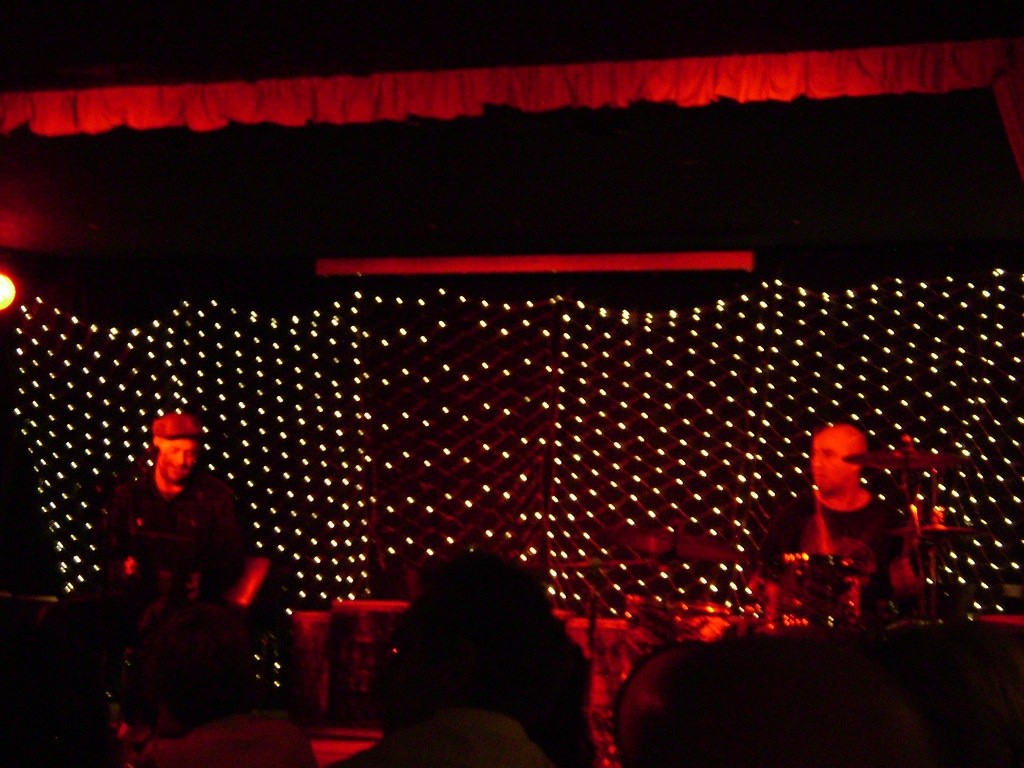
[152,413,206,439]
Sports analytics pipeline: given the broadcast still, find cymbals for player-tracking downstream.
[555,557,633,569]
[605,522,759,573]
[881,518,1000,549]
[831,444,980,476]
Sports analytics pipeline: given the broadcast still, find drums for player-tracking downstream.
[611,632,704,768]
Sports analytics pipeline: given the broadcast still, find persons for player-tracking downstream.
[747,424,920,633]
[99,413,245,595]
[0,513,1024,767]
